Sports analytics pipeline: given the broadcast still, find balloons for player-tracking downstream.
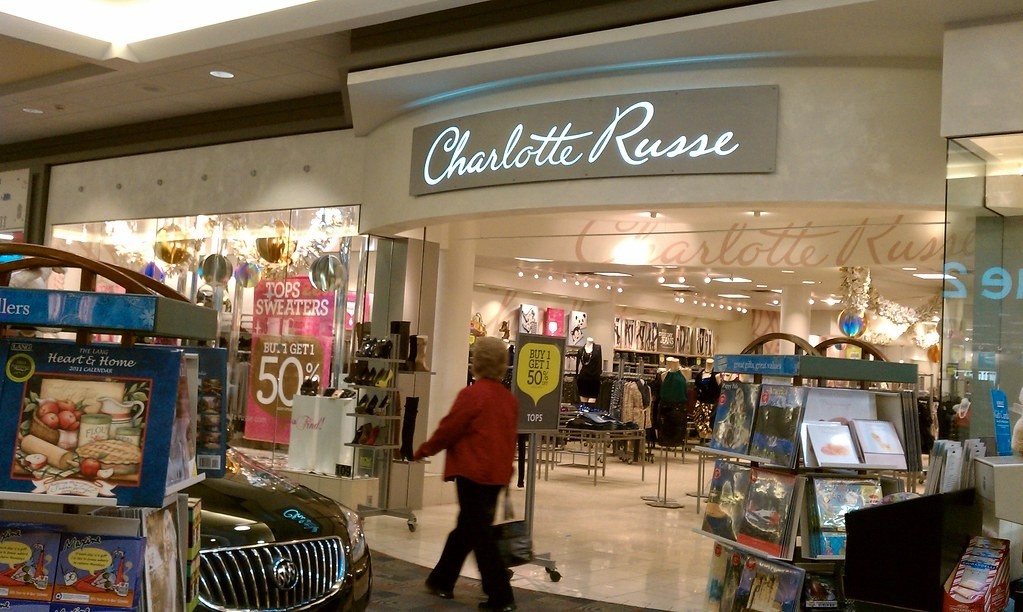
[203,253,234,286]
[936,318,943,337]
[307,254,344,293]
[152,223,189,264]
[836,307,868,338]
[199,260,206,279]
[141,261,165,283]
[235,261,261,289]
[927,344,941,363]
[255,219,298,263]
[834,343,847,350]
[945,317,966,339]
[949,344,964,362]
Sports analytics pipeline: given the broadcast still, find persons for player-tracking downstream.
[409,334,523,612]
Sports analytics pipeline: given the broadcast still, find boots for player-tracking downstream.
[404,333,430,372]
[389,320,411,371]
[394,389,417,464]
[356,322,371,350]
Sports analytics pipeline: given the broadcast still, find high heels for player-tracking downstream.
[355,338,393,359]
[299,379,319,396]
[350,423,389,446]
[343,362,394,388]
[354,393,392,416]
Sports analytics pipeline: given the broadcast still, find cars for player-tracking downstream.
[177,444,373,612]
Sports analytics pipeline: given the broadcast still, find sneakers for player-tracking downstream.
[477,598,518,612]
[425,577,455,599]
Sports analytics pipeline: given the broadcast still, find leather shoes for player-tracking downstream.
[323,387,356,398]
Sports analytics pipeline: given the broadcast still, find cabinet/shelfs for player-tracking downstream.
[508,329,1023,611]
[345,354,437,533]
